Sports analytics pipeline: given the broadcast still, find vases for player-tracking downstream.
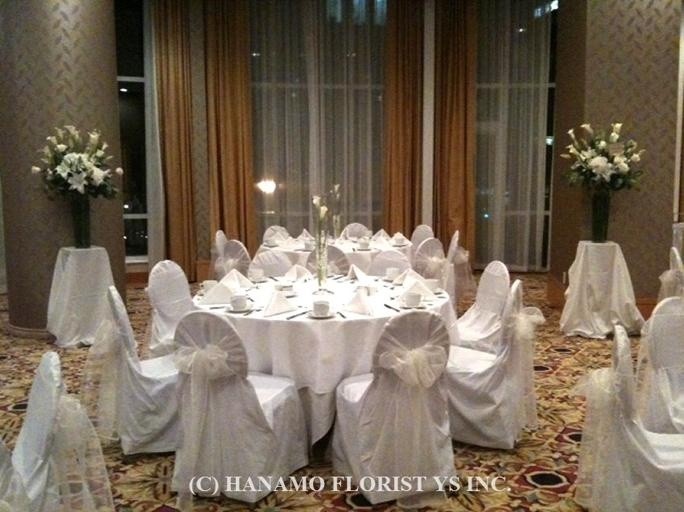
[587,192,613,243]
[66,190,97,249]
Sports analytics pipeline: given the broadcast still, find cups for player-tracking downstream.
[279,228,404,249]
[196,267,428,319]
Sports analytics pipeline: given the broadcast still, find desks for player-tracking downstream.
[41,246,114,350]
[558,240,645,340]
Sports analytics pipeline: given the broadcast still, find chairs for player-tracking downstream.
[1,346,67,512]
[650,244,684,302]
[568,320,684,512]
[641,293,684,437]
[96,216,549,511]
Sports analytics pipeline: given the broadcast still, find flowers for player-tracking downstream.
[553,117,647,193]
[312,179,342,222]
[29,124,127,201]
